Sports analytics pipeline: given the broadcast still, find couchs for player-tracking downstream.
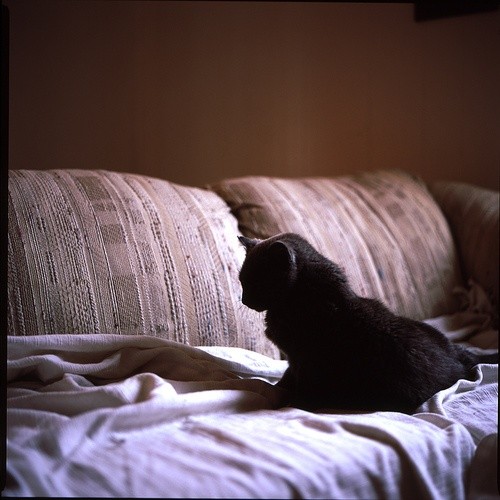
[8,164,500,500]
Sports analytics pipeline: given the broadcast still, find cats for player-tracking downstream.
[235,231,500,416]
